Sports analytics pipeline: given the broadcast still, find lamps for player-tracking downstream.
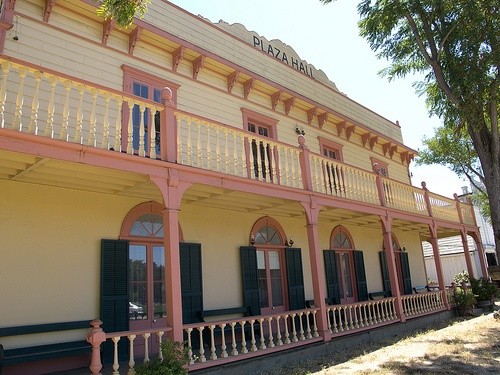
[288,236,294,248]
[250,232,256,246]
[12,14,20,41]
[295,123,306,137]
[402,244,406,252]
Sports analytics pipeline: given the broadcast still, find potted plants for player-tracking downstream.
[450,272,497,316]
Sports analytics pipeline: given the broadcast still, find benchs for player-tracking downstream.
[306,296,348,328]
[368,285,440,314]
